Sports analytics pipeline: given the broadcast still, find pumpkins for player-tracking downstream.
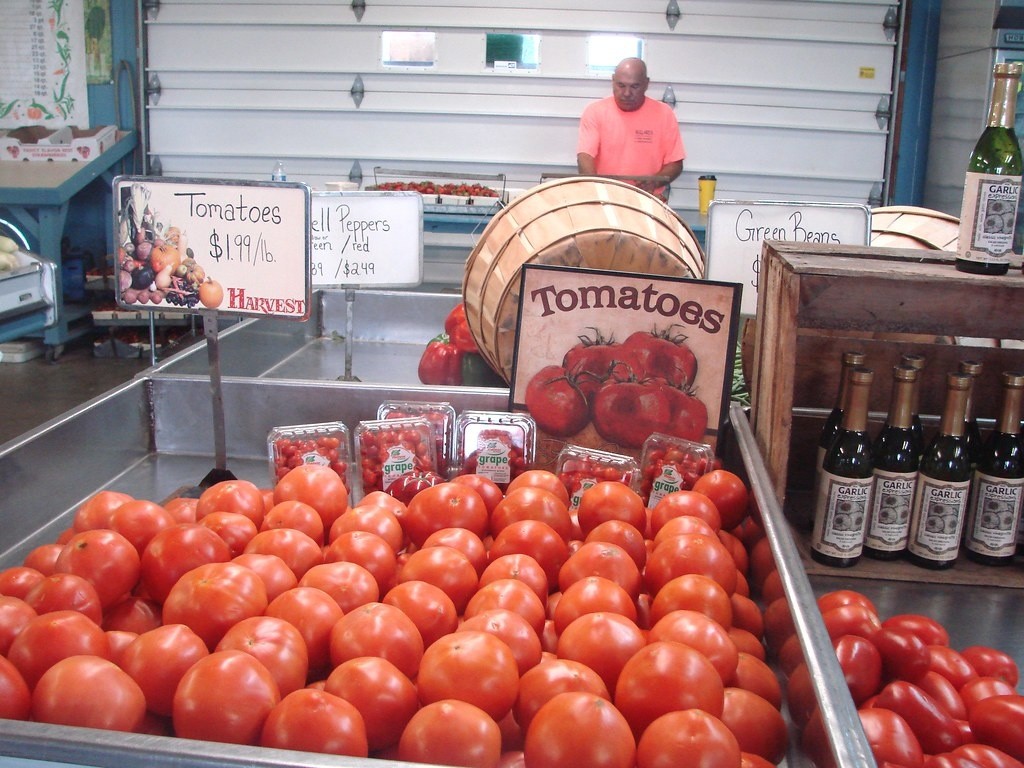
[129,226,224,309]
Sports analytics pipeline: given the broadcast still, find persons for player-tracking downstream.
[576,57,688,205]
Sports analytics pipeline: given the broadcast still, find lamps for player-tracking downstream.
[661,85,676,108]
[352,0,366,21]
[351,75,365,107]
[666,0,680,30]
[351,160,362,189]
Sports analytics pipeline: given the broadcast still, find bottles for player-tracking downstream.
[955,64,1024,274]
[272,161,286,181]
[809,350,1024,568]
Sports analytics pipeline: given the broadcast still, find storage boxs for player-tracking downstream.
[423,194,440,205]
[439,193,469,205]
[471,189,498,207]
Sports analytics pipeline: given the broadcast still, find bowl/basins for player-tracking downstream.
[267,420,352,503]
[353,417,436,505]
[325,182,359,192]
[639,433,715,507]
[453,409,539,497]
[377,399,456,482]
[556,445,642,523]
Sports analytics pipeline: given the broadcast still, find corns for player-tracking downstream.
[0,236,22,270]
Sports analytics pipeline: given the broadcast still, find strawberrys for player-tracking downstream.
[365,179,501,195]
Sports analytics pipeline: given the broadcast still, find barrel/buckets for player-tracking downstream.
[461,175,706,386]
[740,205,1024,396]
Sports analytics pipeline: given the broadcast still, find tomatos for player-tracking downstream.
[0,411,1024,768]
[85,267,194,346]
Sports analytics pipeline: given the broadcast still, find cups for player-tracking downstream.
[698,175,717,214]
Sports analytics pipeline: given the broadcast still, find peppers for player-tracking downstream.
[418,304,502,386]
